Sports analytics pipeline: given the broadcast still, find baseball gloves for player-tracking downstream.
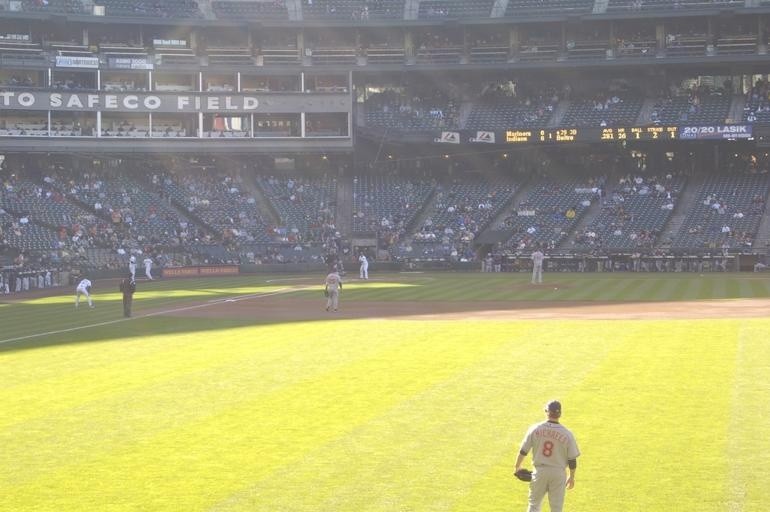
[514,470,531,481]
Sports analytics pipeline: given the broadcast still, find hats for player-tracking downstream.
[545,400,561,412]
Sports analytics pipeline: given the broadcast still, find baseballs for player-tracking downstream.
[554,288,557,290]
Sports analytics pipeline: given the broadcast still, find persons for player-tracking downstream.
[3,0,770,297]
[119,271,137,318]
[514,399,580,512]
[74,275,94,308]
[323,267,343,311]
